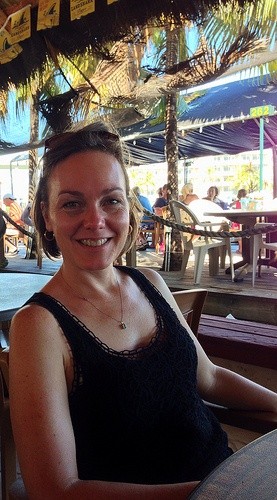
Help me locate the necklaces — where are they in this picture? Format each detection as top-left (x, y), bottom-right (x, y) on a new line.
top-left (60, 266), bottom-right (127, 329)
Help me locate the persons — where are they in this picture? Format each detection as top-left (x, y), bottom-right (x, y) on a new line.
top-left (3, 193), bottom-right (26, 256)
top-left (9, 126), bottom-right (276, 500)
top-left (0, 214), bottom-right (9, 269)
top-left (151, 184), bottom-right (249, 254)
top-left (131, 187), bottom-right (153, 252)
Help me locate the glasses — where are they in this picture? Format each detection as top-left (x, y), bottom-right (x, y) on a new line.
top-left (44, 131), bottom-right (119, 155)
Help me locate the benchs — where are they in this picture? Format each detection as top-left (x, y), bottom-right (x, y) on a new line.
top-left (197, 314), bottom-right (277, 394)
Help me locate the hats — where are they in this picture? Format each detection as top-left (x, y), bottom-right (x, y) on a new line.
top-left (3, 193), bottom-right (16, 200)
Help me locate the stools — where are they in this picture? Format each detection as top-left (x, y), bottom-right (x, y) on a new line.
top-left (3, 236), bottom-right (19, 253)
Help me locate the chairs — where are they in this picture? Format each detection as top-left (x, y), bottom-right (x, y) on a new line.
top-left (169, 200), bottom-right (277, 285)
top-left (140, 208), bottom-right (163, 248)
top-left (0, 288), bottom-right (208, 500)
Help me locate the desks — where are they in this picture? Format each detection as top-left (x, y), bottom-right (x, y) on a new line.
top-left (188, 427), bottom-right (276, 500)
top-left (203, 211), bottom-right (277, 282)
